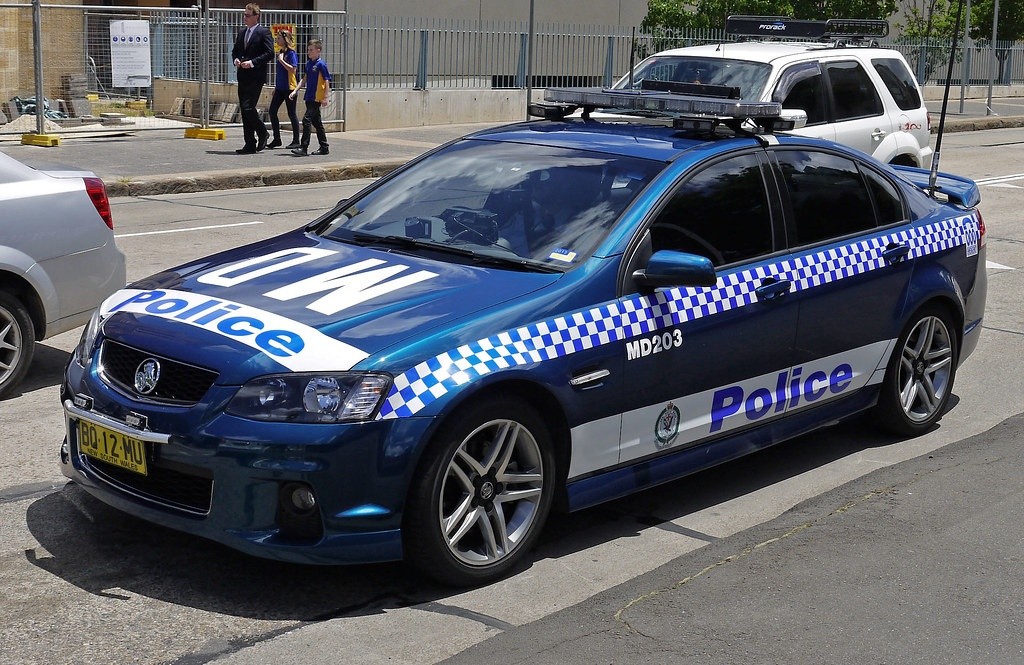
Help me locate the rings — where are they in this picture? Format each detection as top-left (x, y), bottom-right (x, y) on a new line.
top-left (245, 64), bottom-right (247, 65)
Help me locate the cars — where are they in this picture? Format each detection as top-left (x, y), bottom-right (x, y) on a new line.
top-left (59, 83), bottom-right (988, 588)
top-left (0, 151), bottom-right (127, 399)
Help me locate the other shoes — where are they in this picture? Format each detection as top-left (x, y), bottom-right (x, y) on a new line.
top-left (311, 147), bottom-right (329, 155)
top-left (286, 142), bottom-right (300, 149)
top-left (266, 139), bottom-right (282, 148)
top-left (236, 141), bottom-right (256, 154)
top-left (291, 148), bottom-right (307, 156)
top-left (256, 132), bottom-right (270, 151)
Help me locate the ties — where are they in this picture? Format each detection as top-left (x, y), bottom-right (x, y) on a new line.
top-left (244, 29), bottom-right (251, 49)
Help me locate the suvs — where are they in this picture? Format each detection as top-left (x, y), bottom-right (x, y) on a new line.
top-left (565, 16), bottom-right (933, 190)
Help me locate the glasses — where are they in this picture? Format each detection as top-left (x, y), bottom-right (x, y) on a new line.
top-left (245, 13), bottom-right (254, 17)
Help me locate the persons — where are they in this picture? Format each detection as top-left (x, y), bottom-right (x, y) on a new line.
top-left (499, 185), bottom-right (573, 254)
top-left (232, 3), bottom-right (275, 154)
top-left (289, 39), bottom-right (331, 156)
top-left (266, 29), bottom-right (301, 149)
